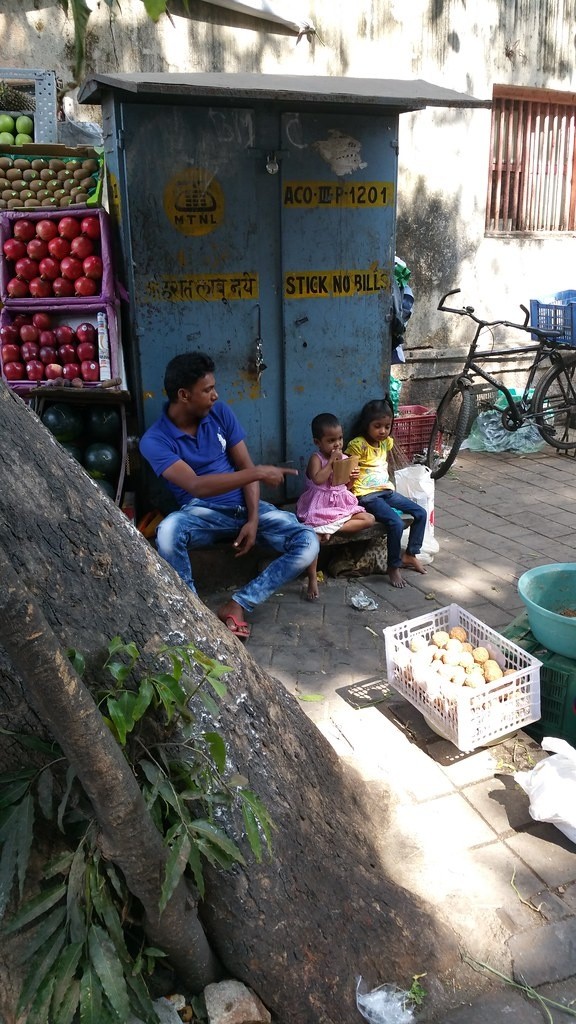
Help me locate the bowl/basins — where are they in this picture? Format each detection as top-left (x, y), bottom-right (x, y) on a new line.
top-left (517, 562), bottom-right (575, 659)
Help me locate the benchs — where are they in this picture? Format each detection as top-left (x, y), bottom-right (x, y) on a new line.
top-left (280, 504), bottom-right (415, 547)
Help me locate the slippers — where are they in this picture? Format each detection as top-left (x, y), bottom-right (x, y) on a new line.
top-left (222, 614), bottom-right (250, 638)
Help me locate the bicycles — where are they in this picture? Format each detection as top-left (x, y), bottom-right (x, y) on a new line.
top-left (426, 287), bottom-right (575, 481)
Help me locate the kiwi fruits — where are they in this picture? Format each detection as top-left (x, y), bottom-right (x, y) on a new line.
top-left (0, 154), bottom-right (101, 214)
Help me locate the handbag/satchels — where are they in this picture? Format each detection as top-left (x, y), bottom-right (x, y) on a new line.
top-left (393, 465), bottom-right (440, 566)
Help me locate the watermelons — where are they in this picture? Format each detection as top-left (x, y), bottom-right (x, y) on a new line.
top-left (29, 395), bottom-right (122, 504)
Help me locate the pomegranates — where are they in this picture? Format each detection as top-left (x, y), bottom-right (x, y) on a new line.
top-left (3, 216), bottom-right (104, 302)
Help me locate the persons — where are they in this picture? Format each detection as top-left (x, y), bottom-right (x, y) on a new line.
top-left (344, 391), bottom-right (428, 588)
top-left (295, 412), bottom-right (376, 599)
top-left (139, 350), bottom-right (321, 638)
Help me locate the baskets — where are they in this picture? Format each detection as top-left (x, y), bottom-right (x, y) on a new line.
top-left (390, 404), bottom-right (443, 462)
top-left (529, 289), bottom-right (576, 346)
top-left (381, 601), bottom-right (543, 752)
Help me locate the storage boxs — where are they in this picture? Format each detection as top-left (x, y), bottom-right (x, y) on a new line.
top-left (528, 289), bottom-right (576, 349)
top-left (498, 609), bottom-right (576, 749)
top-left (0, 141), bottom-right (106, 210)
top-left (0, 304), bottom-right (118, 388)
top-left (40, 393), bottom-right (128, 512)
top-left (391, 405), bottom-right (443, 463)
top-left (0, 209), bottom-right (117, 306)
top-left (383, 604), bottom-right (543, 753)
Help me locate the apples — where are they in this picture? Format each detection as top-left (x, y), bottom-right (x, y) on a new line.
top-left (1, 313), bottom-right (103, 383)
top-left (0, 110), bottom-right (32, 145)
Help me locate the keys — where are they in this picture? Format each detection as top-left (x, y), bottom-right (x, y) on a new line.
top-left (252, 338), bottom-right (267, 382)
